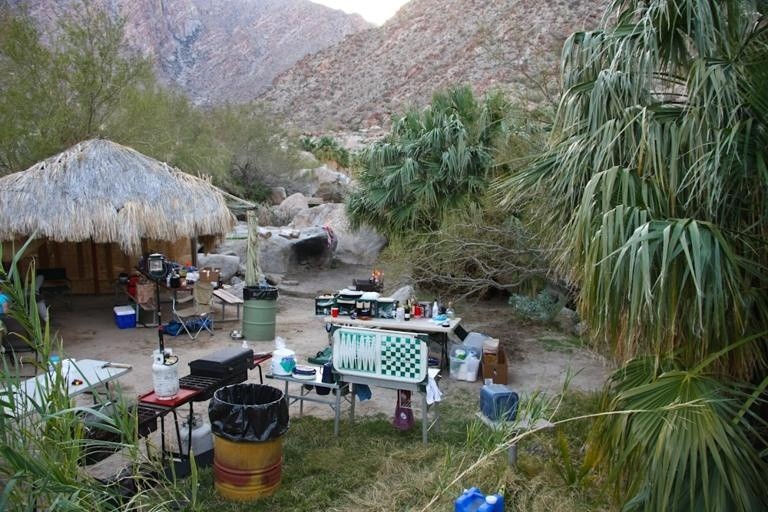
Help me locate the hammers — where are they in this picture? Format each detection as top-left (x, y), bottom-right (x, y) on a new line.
top-left (101, 361), bottom-right (132, 369)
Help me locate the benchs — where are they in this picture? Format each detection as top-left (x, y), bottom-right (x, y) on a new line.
top-left (116, 284), bottom-right (159, 327)
top-left (213, 288), bottom-right (243, 320)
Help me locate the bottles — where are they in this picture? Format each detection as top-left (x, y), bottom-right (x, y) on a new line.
top-left (405, 297), bottom-right (443, 322)
top-left (445, 301), bottom-right (455, 321)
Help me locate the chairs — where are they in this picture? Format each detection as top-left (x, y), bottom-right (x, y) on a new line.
top-left (169, 282), bottom-right (214, 340)
top-left (36, 266), bottom-right (72, 313)
top-left (0, 309), bottom-right (45, 378)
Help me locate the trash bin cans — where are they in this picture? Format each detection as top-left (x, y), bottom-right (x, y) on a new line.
top-left (242, 286), bottom-right (279, 341)
top-left (208, 384), bottom-right (289, 502)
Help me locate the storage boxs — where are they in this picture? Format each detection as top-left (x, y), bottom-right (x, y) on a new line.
top-left (113, 305), bottom-right (135, 328)
top-left (448, 332), bottom-right (511, 386)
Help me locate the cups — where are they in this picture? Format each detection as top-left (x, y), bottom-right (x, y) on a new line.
top-left (393, 308), bottom-right (404, 323)
top-left (49, 356), bottom-right (75, 388)
top-left (331, 308), bottom-right (339, 318)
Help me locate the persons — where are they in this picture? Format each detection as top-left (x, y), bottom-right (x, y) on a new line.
top-left (0, 285), bottom-right (12, 315)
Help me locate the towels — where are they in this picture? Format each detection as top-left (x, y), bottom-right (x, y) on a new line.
top-left (425, 373), bottom-right (441, 405)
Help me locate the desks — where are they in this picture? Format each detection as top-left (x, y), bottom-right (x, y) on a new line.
top-left (135, 263), bottom-right (192, 327)
top-left (0, 359), bottom-right (133, 424)
top-left (325, 312), bottom-right (463, 374)
top-left (324, 325), bottom-right (441, 442)
top-left (264, 365), bottom-right (357, 436)
